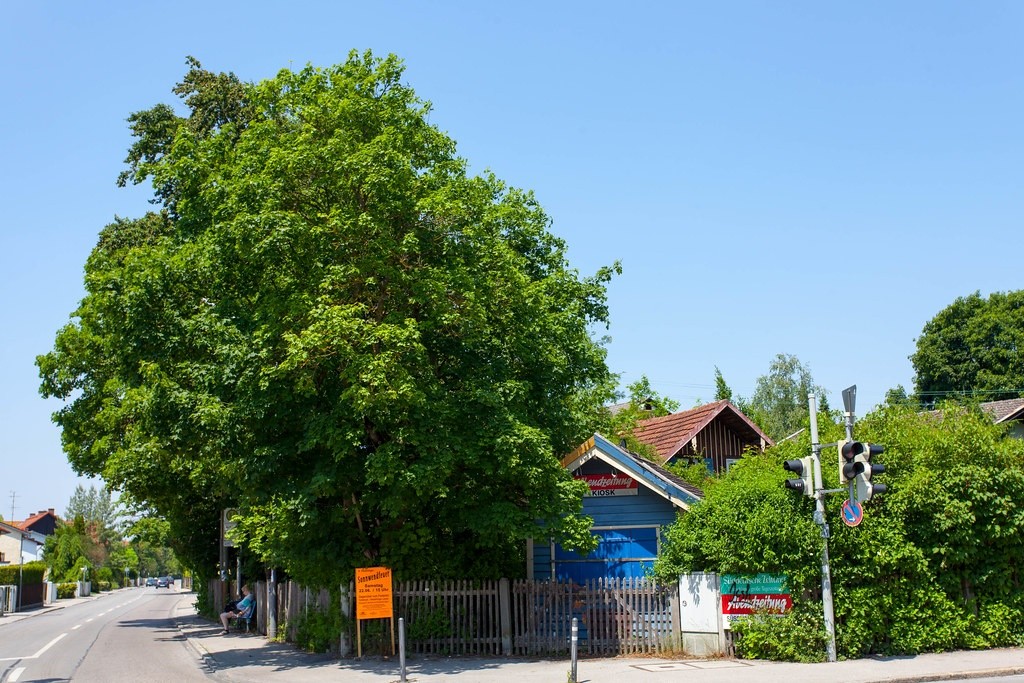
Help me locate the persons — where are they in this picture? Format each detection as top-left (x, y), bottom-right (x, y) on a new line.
top-left (220, 585), bottom-right (254, 636)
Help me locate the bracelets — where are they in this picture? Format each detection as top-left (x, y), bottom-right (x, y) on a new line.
top-left (240, 612), bottom-right (242, 614)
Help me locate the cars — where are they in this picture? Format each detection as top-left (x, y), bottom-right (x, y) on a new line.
top-left (146, 578), bottom-right (155, 587)
top-left (166, 575), bottom-right (175, 585)
top-left (156, 577), bottom-right (170, 589)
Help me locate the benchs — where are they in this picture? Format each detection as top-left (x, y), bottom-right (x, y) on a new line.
top-left (231, 601), bottom-right (257, 638)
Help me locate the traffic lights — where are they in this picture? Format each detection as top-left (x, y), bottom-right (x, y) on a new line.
top-left (783, 456), bottom-right (813, 496)
top-left (854, 442), bottom-right (888, 501)
top-left (837, 440), bottom-right (864, 484)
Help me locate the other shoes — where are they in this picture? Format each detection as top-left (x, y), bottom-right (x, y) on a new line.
top-left (219, 630), bottom-right (229, 635)
